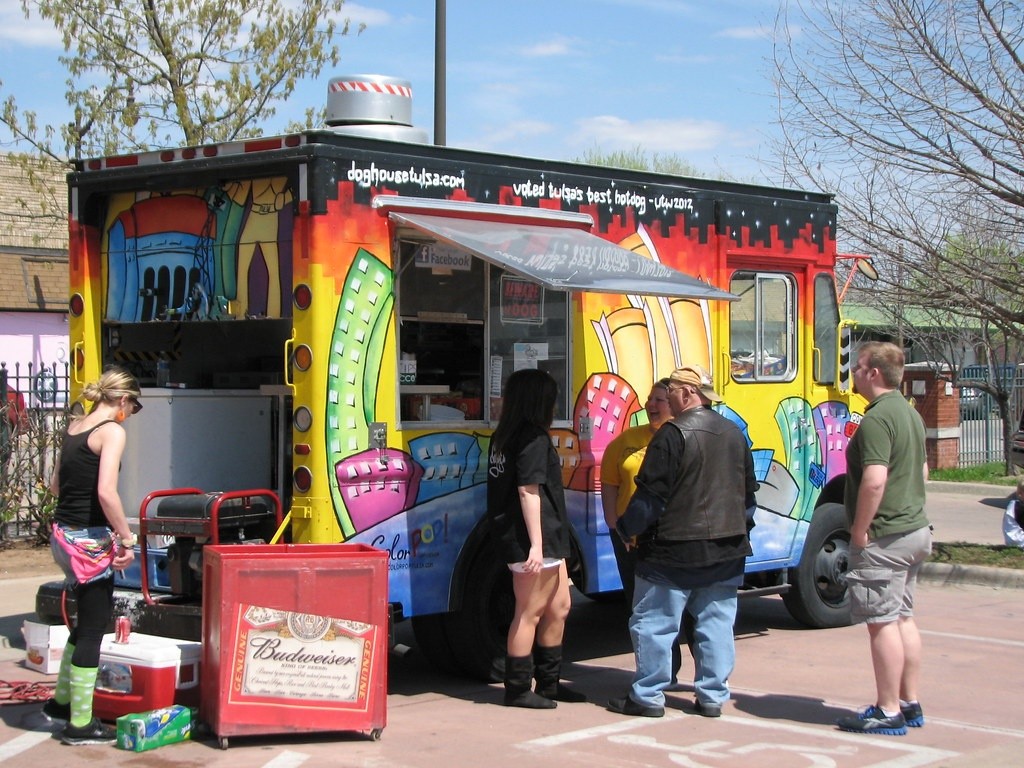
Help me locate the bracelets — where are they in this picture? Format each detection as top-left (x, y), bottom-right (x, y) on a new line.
top-left (121, 534), bottom-right (137, 548)
top-left (122, 545), bottom-right (135, 550)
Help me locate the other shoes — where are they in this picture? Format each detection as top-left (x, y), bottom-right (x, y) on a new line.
top-left (695, 695), bottom-right (721, 717)
top-left (607, 697), bottom-right (664, 716)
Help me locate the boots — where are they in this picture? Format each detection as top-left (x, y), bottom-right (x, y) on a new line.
top-left (534, 645), bottom-right (586, 703)
top-left (505, 654), bottom-right (557, 710)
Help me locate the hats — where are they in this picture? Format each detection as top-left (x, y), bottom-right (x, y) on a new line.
top-left (671, 364), bottom-right (723, 404)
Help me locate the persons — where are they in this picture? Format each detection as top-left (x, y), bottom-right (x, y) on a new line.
top-left (599, 379), bottom-right (694, 690)
top-left (487, 369), bottom-right (586, 708)
top-left (607, 364), bottom-right (757, 717)
top-left (835, 342), bottom-right (931, 736)
top-left (39, 368), bottom-right (139, 745)
top-left (1002, 480), bottom-right (1024, 548)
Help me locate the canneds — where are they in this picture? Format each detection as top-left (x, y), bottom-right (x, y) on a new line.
top-left (114, 616), bottom-right (131, 644)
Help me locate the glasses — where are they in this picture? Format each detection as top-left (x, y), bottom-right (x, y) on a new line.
top-left (666, 386), bottom-right (696, 396)
top-left (129, 395), bottom-right (144, 415)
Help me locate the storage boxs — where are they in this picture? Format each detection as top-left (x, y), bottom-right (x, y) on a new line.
top-left (20, 542), bottom-right (387, 751)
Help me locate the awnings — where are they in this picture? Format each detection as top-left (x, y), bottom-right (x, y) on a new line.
top-left (387, 212), bottom-right (742, 302)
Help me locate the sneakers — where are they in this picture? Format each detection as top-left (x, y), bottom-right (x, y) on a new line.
top-left (61, 719), bottom-right (119, 746)
top-left (837, 706), bottom-right (906, 735)
top-left (857, 702), bottom-right (924, 727)
top-left (40, 697), bottom-right (70, 724)
